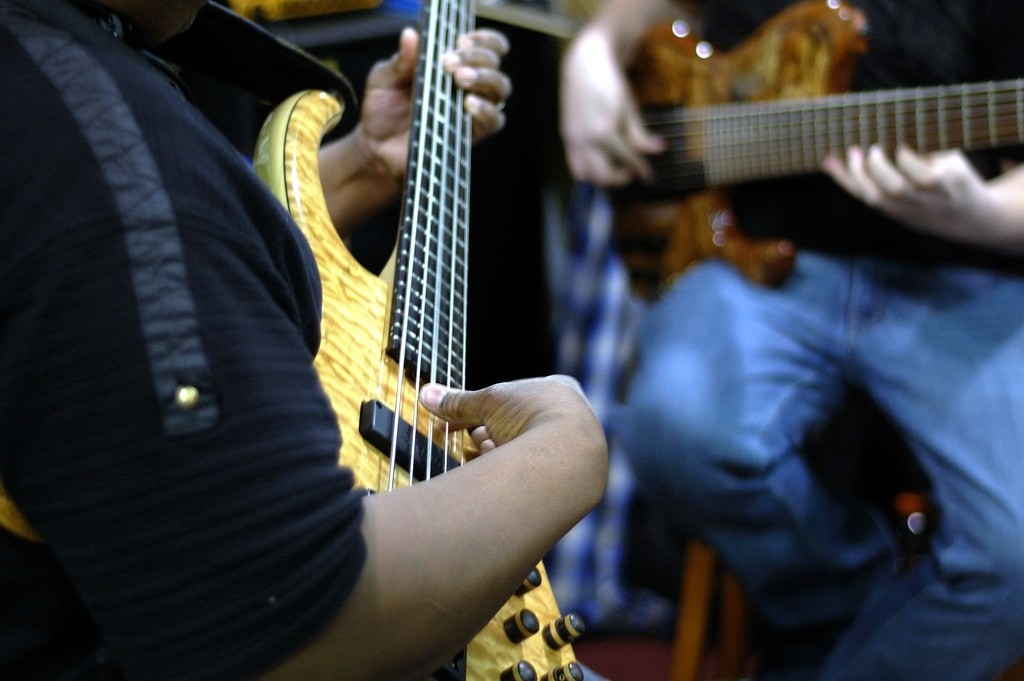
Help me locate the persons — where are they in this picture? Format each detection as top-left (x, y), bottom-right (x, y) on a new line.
top-left (560, 0), bottom-right (1024, 681)
top-left (0, 0), bottom-right (608, 681)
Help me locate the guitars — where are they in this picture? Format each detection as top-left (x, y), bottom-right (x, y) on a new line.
top-left (606, 0), bottom-right (1023, 304)
top-left (250, 0), bottom-right (589, 681)
